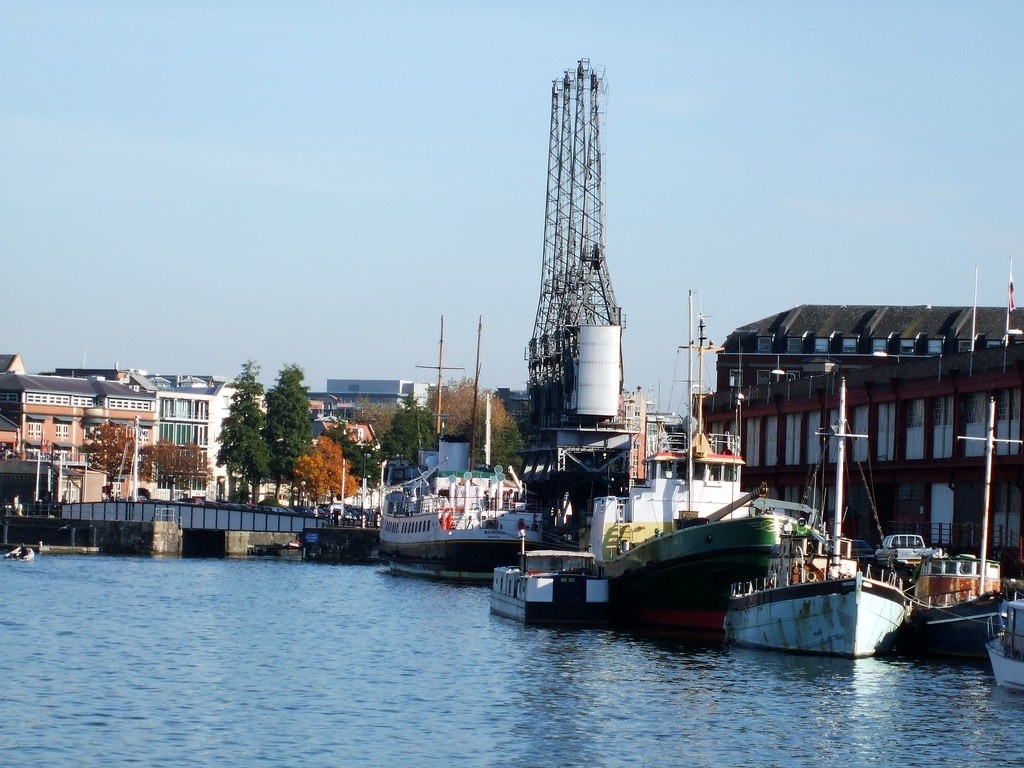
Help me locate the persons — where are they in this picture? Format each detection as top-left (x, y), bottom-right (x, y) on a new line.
top-left (328, 502), bottom-right (335, 523)
top-left (13, 494), bottom-right (19, 515)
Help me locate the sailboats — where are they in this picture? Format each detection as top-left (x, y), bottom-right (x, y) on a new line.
top-left (721, 374), bottom-right (908, 660)
top-left (895, 397), bottom-right (1024, 658)
top-left (587, 290), bottom-right (825, 636)
top-left (376, 313), bottom-right (555, 583)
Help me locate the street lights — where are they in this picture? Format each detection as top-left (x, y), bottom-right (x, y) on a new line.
top-left (361, 452), bottom-right (372, 528)
top-left (301, 480), bottom-right (306, 508)
top-left (219, 478), bottom-right (225, 501)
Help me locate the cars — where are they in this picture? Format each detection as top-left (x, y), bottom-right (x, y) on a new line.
top-left (850, 538), bottom-right (876, 570)
top-left (177, 496), bottom-right (381, 528)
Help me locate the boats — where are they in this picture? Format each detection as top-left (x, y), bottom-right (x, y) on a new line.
top-left (983, 588), bottom-right (1024, 691)
top-left (488, 527), bottom-right (612, 627)
top-left (3, 546), bottom-right (36, 563)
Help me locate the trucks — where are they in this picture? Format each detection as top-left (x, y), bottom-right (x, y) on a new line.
top-left (873, 534), bottom-right (938, 567)
